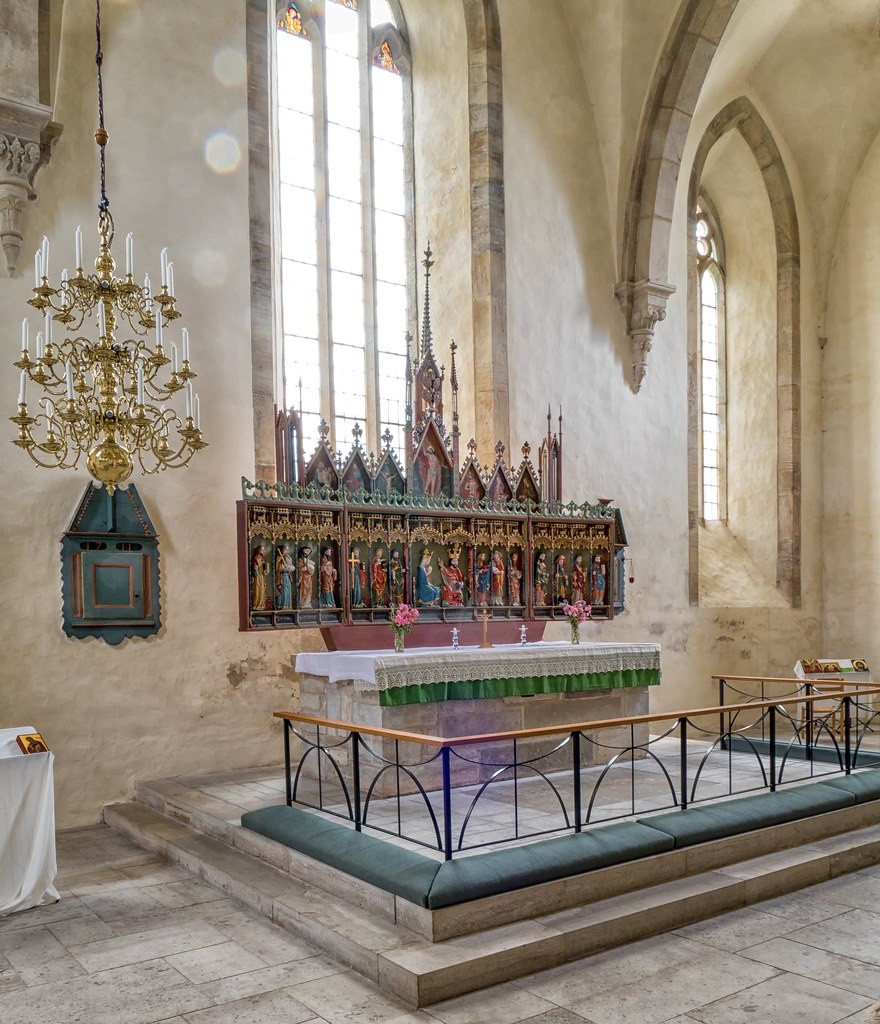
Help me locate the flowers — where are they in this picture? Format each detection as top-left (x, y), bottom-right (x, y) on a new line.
top-left (563, 599), bottom-right (593, 643)
top-left (388, 604), bottom-right (419, 649)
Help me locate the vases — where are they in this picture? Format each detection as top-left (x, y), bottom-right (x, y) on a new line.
top-left (394, 629), bottom-right (405, 653)
top-left (571, 624), bottom-right (579, 645)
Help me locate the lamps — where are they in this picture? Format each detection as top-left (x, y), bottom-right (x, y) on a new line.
top-left (8, 0), bottom-right (209, 497)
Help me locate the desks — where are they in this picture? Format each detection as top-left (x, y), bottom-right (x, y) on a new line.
top-left (0, 728), bottom-right (60, 912)
top-left (795, 658), bottom-right (873, 737)
top-left (295, 640), bottom-right (663, 797)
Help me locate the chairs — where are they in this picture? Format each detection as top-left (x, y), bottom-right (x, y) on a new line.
top-left (800, 677), bottom-right (845, 743)
